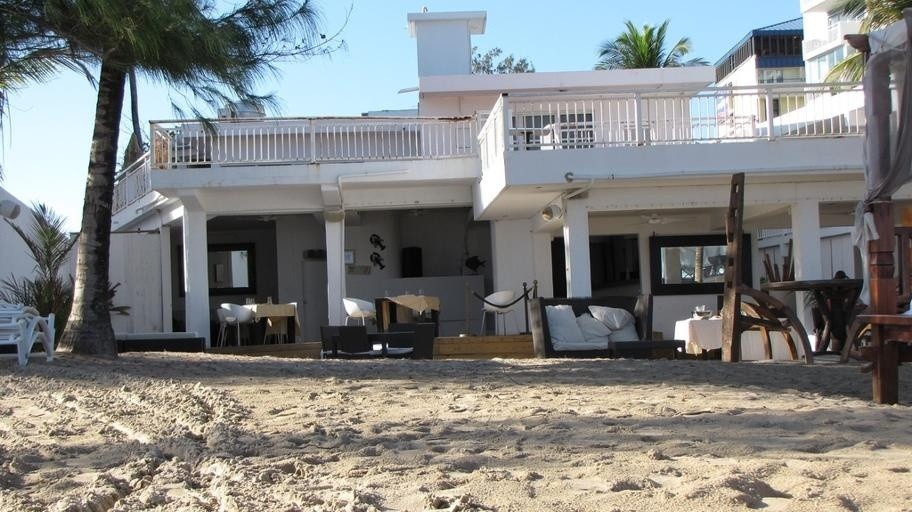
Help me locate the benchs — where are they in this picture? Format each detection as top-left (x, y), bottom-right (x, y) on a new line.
top-left (527, 293), bottom-right (653, 358)
top-left (609, 341), bottom-right (685, 359)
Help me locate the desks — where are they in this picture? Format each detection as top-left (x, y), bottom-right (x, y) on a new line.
top-left (375, 296), bottom-right (441, 336)
top-left (675, 317), bottom-right (796, 358)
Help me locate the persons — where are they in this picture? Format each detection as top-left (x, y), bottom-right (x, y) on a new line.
top-left (815, 270), bottom-right (846, 353)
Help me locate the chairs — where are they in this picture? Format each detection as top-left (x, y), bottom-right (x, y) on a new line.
top-left (321, 323), bottom-right (436, 360)
top-left (342, 297), bottom-right (377, 326)
top-left (0, 300), bottom-right (56, 366)
top-left (218, 302), bottom-right (298, 347)
top-left (481, 290), bottom-right (521, 336)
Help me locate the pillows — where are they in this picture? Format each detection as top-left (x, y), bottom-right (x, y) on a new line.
top-left (545, 304), bottom-right (639, 352)
